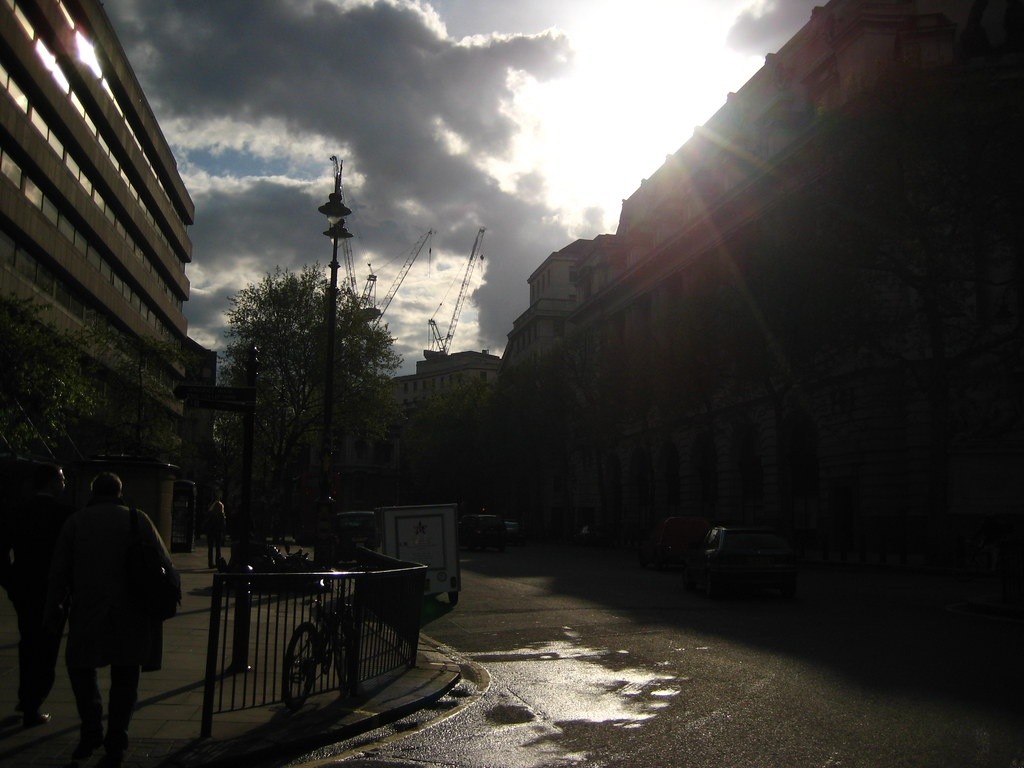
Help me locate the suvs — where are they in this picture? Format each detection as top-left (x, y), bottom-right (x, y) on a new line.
top-left (686, 522), bottom-right (800, 600)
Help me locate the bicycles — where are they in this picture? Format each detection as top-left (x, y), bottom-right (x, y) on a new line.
top-left (280, 579), bottom-right (358, 711)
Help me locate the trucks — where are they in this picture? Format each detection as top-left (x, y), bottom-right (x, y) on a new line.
top-left (373, 503), bottom-right (463, 608)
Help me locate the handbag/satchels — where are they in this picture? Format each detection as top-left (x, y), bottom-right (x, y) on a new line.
top-left (127, 540), bottom-right (180, 621)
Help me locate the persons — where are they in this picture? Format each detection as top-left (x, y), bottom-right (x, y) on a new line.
top-left (0, 458), bottom-right (81, 729)
top-left (46, 470), bottom-right (180, 762)
top-left (205, 500), bottom-right (226, 569)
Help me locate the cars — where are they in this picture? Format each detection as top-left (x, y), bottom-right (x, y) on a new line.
top-left (639, 515), bottom-right (713, 570)
top-left (336, 510), bottom-right (379, 551)
top-left (456, 511), bottom-right (525, 552)
top-left (570, 524), bottom-right (612, 548)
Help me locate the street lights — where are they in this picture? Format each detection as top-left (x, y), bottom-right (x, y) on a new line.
top-left (315, 172), bottom-right (355, 567)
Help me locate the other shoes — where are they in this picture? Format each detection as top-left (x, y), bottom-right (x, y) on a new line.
top-left (23, 716), bottom-right (47, 729)
top-left (98, 755), bottom-right (121, 768)
top-left (71, 731), bottom-right (102, 759)
top-left (14, 702), bottom-right (24, 713)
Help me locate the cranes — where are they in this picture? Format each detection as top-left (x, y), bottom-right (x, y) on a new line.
top-left (425, 320), bottom-right (450, 354)
top-left (444, 226), bottom-right (488, 356)
top-left (334, 228), bottom-right (437, 332)
